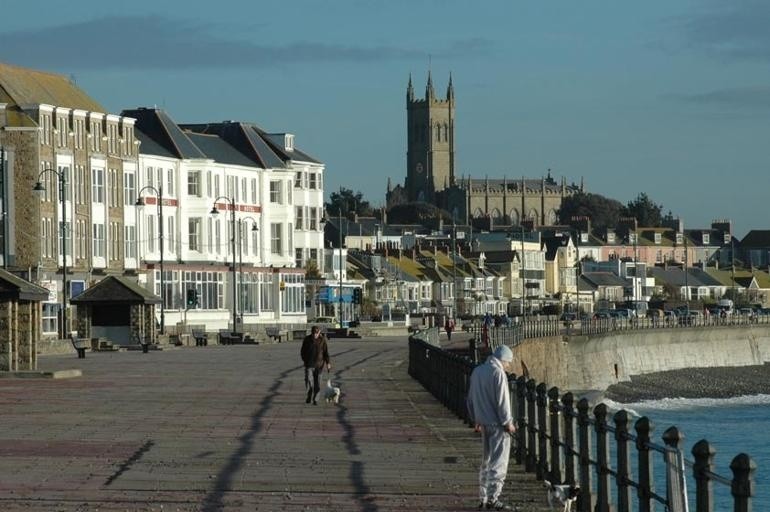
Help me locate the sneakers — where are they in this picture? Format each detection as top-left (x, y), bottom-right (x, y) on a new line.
top-left (477, 498), bottom-right (511, 509)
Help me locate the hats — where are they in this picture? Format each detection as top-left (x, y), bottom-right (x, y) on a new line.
top-left (311, 326), bottom-right (319, 334)
top-left (493, 345), bottom-right (513, 362)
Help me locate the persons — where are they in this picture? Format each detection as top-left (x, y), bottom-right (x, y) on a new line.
top-left (435, 316), bottom-right (441, 336)
top-left (702, 307), bottom-right (711, 326)
top-left (454, 308), bottom-right (519, 363)
top-left (721, 307), bottom-right (727, 325)
top-left (466, 345), bottom-right (516, 512)
top-left (444, 316), bottom-right (455, 340)
top-left (301, 326), bottom-right (331, 406)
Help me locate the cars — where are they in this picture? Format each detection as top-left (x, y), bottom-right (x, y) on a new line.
top-left (561, 299), bottom-right (766, 327)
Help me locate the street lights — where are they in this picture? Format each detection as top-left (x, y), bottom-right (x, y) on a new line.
top-left (320, 201), bottom-right (343, 328)
top-left (31, 169), bottom-right (68, 338)
top-left (210, 196), bottom-right (258, 334)
top-left (187, 288), bottom-right (198, 310)
top-left (136, 183), bottom-right (165, 334)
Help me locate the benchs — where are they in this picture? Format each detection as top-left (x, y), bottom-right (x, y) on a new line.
top-left (68, 335), bottom-right (91, 360)
top-left (190, 327), bottom-right (241, 346)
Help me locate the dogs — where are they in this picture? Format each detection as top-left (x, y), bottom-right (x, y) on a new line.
top-left (324, 379), bottom-right (340, 404)
top-left (544, 480), bottom-right (581, 512)
